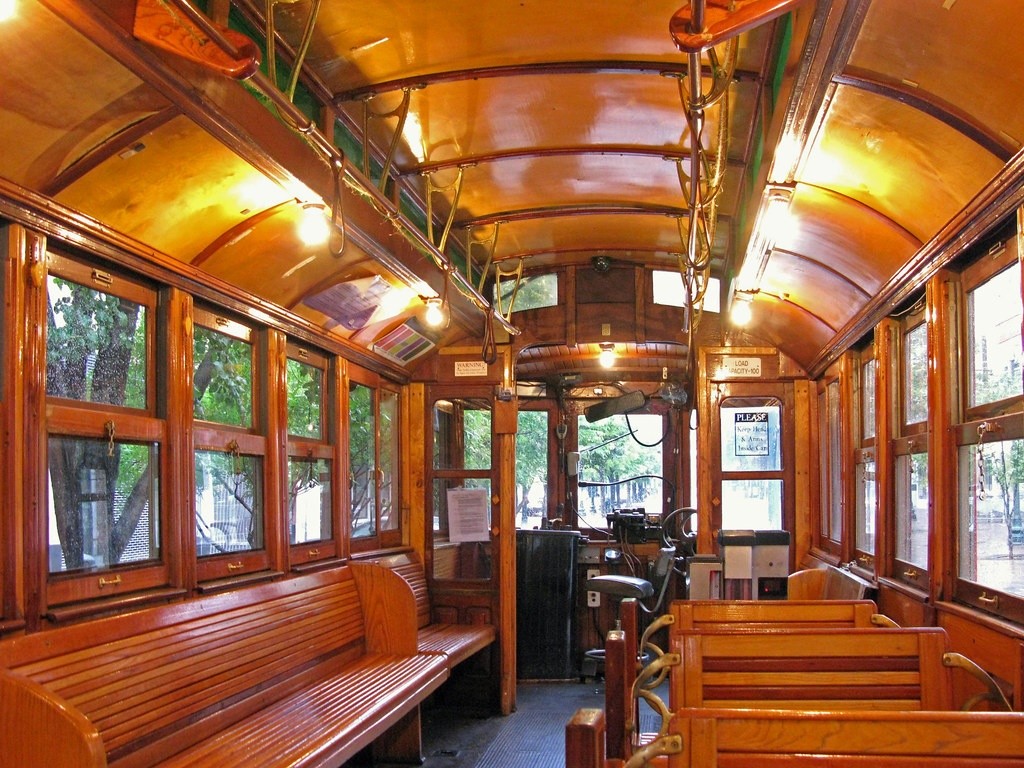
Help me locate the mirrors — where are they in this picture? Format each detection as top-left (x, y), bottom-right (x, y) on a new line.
top-left (584, 390), bottom-right (646, 423)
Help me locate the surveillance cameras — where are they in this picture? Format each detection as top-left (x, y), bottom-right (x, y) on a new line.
top-left (592, 257), bottom-right (610, 272)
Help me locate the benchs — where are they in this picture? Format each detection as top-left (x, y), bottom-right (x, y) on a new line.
top-left (0, 551), bottom-right (500, 768)
top-left (565, 567), bottom-right (1024, 768)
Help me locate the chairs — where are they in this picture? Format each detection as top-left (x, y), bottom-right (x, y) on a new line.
top-left (583, 547), bottom-right (678, 683)
